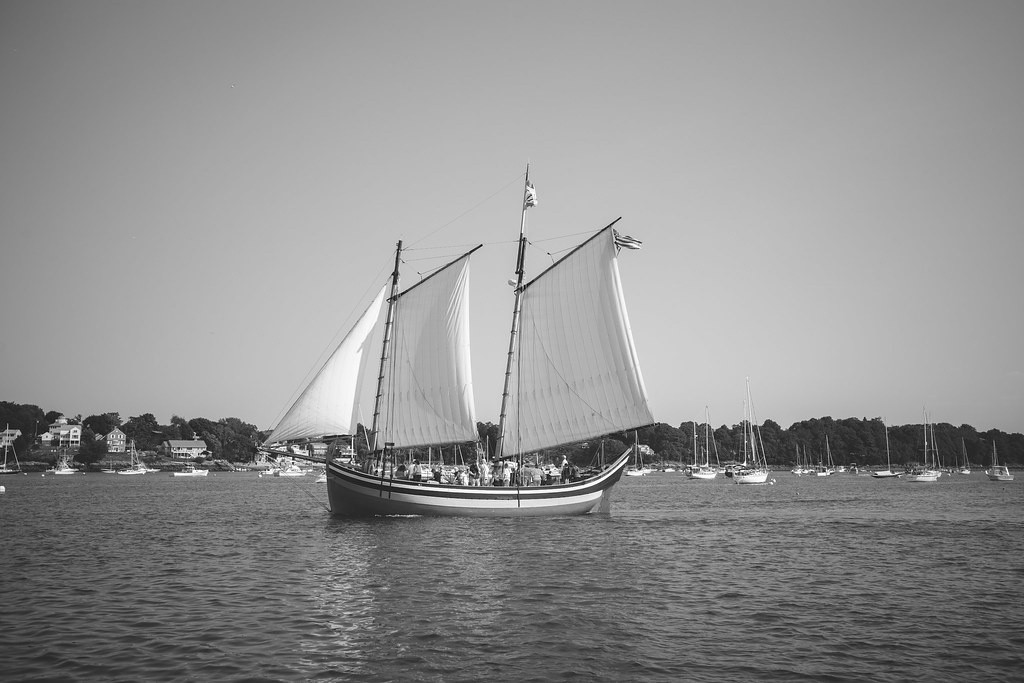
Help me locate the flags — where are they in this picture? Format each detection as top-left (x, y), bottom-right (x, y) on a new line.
top-left (613, 228), bottom-right (642, 250)
top-left (525, 184), bottom-right (537, 207)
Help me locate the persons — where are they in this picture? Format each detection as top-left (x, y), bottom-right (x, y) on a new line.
top-left (395, 455), bottom-right (580, 487)
top-left (737, 470), bottom-right (754, 475)
top-left (693, 468), bottom-right (701, 474)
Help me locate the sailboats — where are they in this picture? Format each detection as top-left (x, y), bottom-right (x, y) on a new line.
top-left (259, 159), bottom-right (654, 519)
top-left (0, 378), bottom-right (1015, 485)
